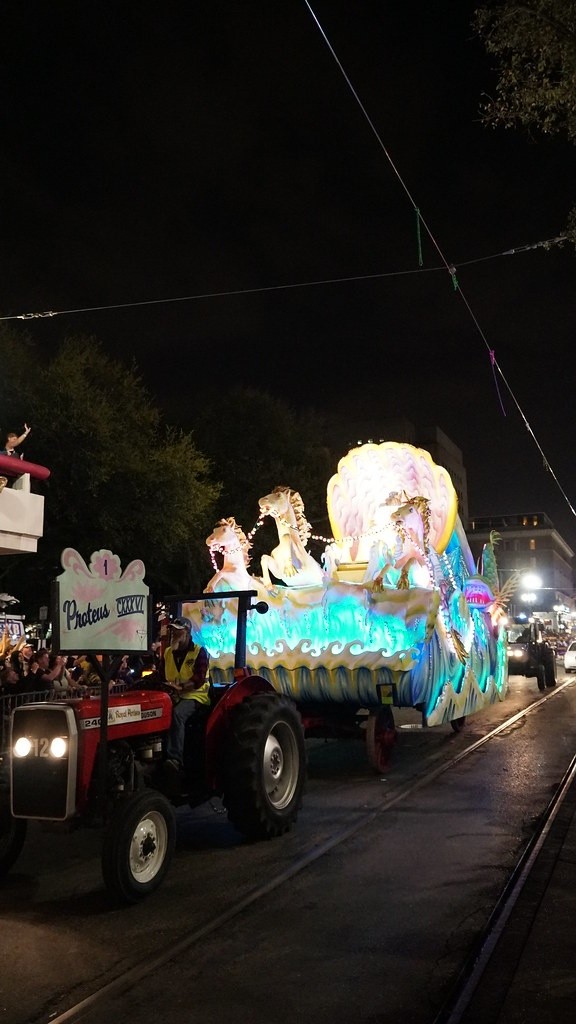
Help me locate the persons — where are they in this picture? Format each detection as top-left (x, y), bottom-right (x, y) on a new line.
top-left (0, 625), bottom-right (167, 722)
top-left (142, 616), bottom-right (208, 772)
top-left (539, 628), bottom-right (576, 655)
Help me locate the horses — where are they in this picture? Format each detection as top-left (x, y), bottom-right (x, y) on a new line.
top-left (376, 490), bottom-right (435, 589)
top-left (202, 516), bottom-right (265, 591)
top-left (258, 485), bottom-right (326, 587)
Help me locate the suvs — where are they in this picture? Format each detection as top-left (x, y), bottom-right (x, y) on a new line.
top-left (504, 615), bottom-right (558, 689)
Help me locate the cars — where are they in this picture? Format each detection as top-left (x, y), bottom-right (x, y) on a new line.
top-left (563, 641), bottom-right (576, 673)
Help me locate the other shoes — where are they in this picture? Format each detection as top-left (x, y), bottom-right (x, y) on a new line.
top-left (166, 759), bottom-right (179, 772)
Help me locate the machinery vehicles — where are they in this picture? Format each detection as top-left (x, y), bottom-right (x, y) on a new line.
top-left (1, 590), bottom-right (468, 906)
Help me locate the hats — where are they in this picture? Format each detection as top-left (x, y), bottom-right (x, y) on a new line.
top-left (166, 617), bottom-right (192, 633)
top-left (5, 644), bottom-right (17, 653)
